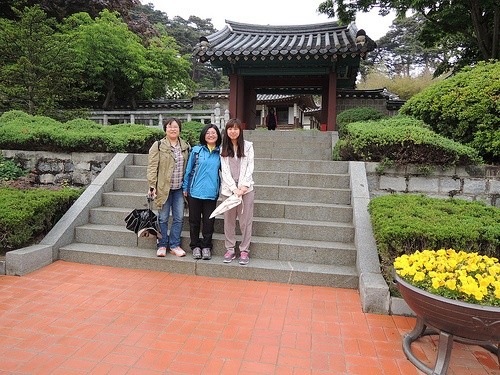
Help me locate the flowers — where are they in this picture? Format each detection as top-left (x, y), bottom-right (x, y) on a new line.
top-left (393, 248), bottom-right (500, 307)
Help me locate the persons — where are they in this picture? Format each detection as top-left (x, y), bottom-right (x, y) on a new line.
top-left (219, 118), bottom-right (254, 264)
top-left (265, 108), bottom-right (277, 130)
top-left (146, 118), bottom-right (191, 257)
top-left (183, 124), bottom-right (222, 259)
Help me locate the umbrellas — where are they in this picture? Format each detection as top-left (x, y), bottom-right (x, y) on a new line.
top-left (124, 187), bottom-right (162, 239)
top-left (209, 193), bottom-right (242, 218)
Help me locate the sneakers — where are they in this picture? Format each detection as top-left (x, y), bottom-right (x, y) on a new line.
top-left (202, 248), bottom-right (211, 260)
top-left (239, 252), bottom-right (249, 264)
top-left (168, 246), bottom-right (186, 256)
top-left (192, 247), bottom-right (201, 258)
top-left (223, 250), bottom-right (235, 263)
top-left (156, 247), bottom-right (166, 256)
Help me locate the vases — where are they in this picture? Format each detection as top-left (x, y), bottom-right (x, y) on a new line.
top-left (395, 274), bottom-right (500, 342)
